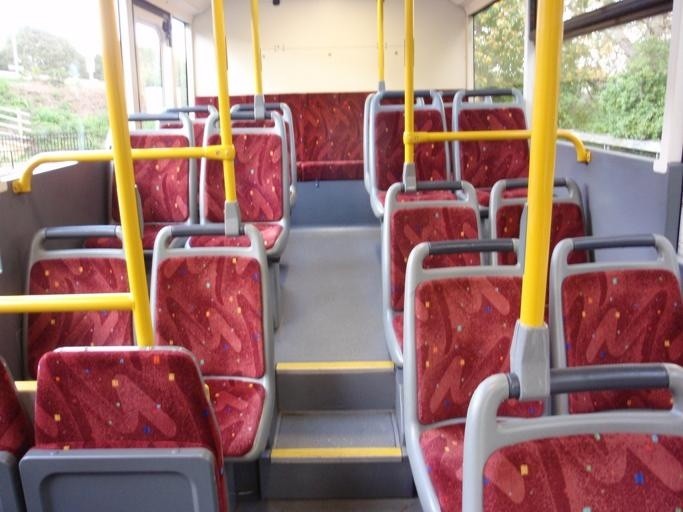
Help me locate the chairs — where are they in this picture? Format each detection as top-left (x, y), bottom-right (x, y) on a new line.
top-left (0, 91), bottom-right (683, 512)
